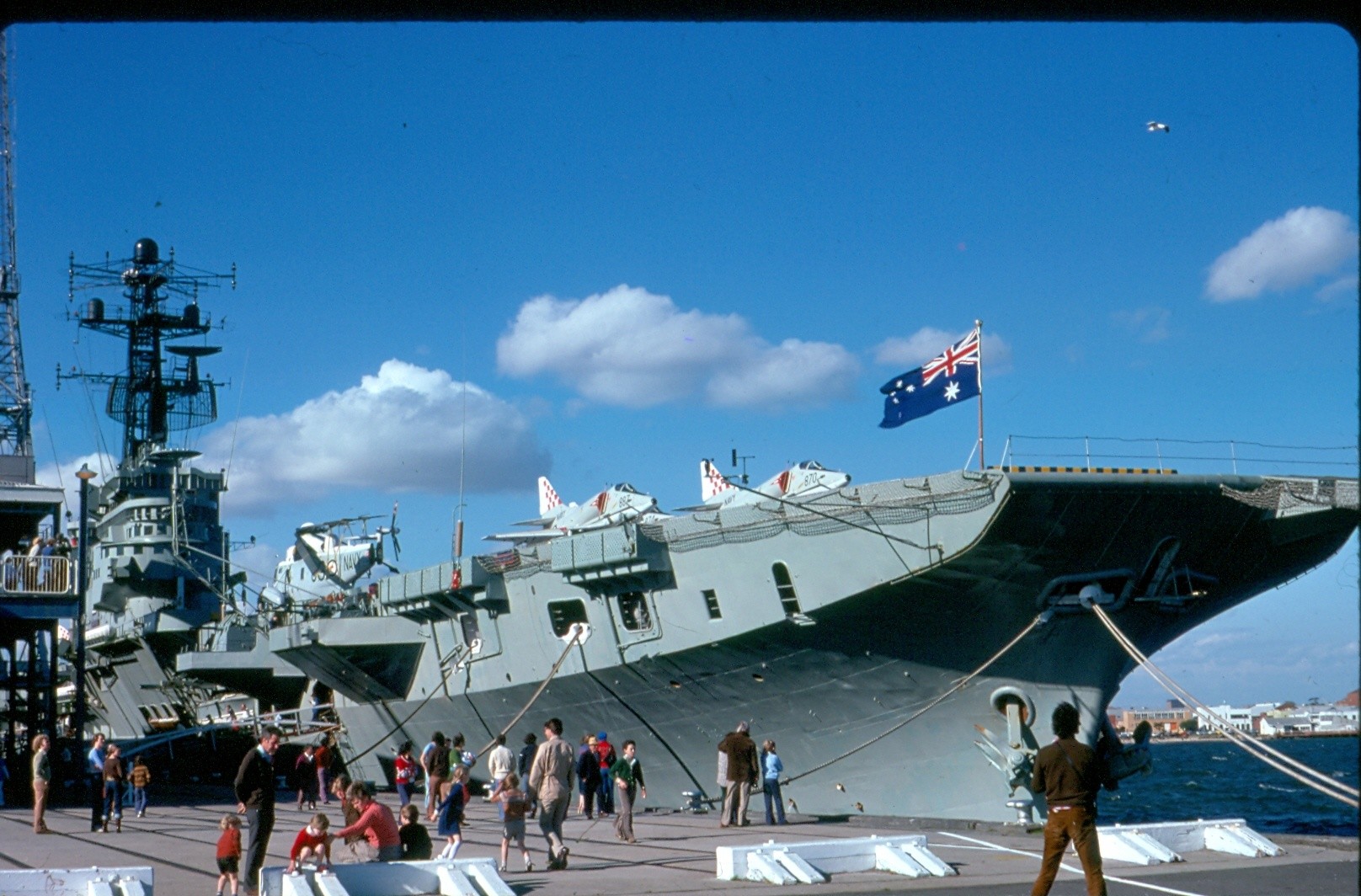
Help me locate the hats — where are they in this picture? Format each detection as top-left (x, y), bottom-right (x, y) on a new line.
top-left (599, 731), bottom-right (607, 740)
top-left (589, 736), bottom-right (600, 745)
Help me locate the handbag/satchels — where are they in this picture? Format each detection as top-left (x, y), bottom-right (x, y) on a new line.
top-left (1092, 794), bottom-right (1099, 818)
top-left (605, 752), bottom-right (617, 767)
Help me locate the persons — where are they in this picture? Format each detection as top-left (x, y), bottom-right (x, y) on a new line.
top-left (608, 740), bottom-right (646, 843)
top-left (285, 813), bottom-right (333, 876)
top-left (716, 732), bottom-right (740, 825)
top-left (527, 718), bottom-right (577, 872)
top-left (398, 805), bottom-right (432, 860)
top-left (101, 743), bottom-right (124, 832)
top-left (88, 733), bottom-right (104, 832)
top-left (1030, 702), bottom-right (1108, 896)
top-left (256, 595), bottom-right (332, 627)
top-left (717, 720), bottom-right (759, 826)
top-left (326, 781), bottom-right (402, 863)
top-left (32, 734), bottom-right (54, 833)
top-left (429, 764), bottom-right (469, 859)
top-left (489, 773), bottom-right (534, 872)
top-left (216, 815), bottom-right (241, 896)
top-left (331, 774), bottom-right (375, 864)
top-left (0, 533), bottom-right (71, 594)
top-left (0, 694), bottom-right (617, 822)
top-left (234, 726), bottom-right (283, 896)
top-left (760, 740), bottom-right (788, 825)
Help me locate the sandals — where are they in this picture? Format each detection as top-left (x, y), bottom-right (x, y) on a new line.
top-left (525, 860), bottom-right (532, 872)
top-left (497, 865), bottom-right (507, 871)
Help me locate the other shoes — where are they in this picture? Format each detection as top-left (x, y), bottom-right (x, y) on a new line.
top-left (92, 826), bottom-right (104, 832)
top-left (137, 814), bottom-right (143, 818)
top-left (617, 833), bottom-right (627, 841)
top-left (628, 836), bottom-right (636, 843)
top-left (138, 811), bottom-right (145, 816)
top-left (247, 889), bottom-right (258, 896)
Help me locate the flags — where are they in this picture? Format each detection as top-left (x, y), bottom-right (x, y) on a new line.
top-left (879, 328), bottom-right (980, 429)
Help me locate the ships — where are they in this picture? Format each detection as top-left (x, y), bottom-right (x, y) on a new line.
top-left (3, 237), bottom-right (1360, 826)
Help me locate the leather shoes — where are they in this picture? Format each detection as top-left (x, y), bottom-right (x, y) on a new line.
top-left (559, 848), bottom-right (570, 869)
top-left (547, 861), bottom-right (559, 869)
top-left (36, 829), bottom-right (54, 834)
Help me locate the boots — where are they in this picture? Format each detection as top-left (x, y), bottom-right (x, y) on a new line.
top-left (116, 818), bottom-right (121, 832)
top-left (103, 820), bottom-right (108, 833)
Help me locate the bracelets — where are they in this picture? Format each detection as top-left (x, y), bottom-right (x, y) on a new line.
top-left (642, 787), bottom-right (645, 789)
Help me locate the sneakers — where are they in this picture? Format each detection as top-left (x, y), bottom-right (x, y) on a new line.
top-left (316, 865), bottom-right (330, 874)
top-left (293, 866), bottom-right (303, 877)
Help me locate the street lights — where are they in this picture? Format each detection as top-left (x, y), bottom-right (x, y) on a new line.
top-left (74, 462), bottom-right (97, 805)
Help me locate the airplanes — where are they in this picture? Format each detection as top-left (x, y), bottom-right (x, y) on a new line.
top-left (672, 457), bottom-right (850, 512)
top-left (481, 475), bottom-right (658, 546)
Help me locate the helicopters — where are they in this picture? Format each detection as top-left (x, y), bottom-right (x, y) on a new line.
top-left (256, 501), bottom-right (403, 624)
